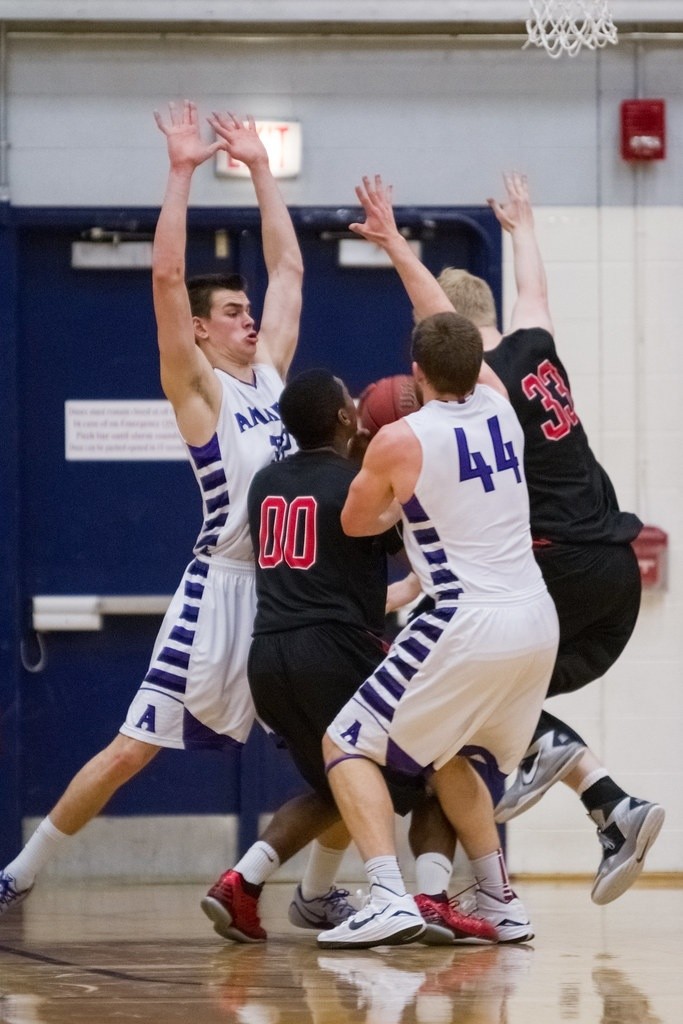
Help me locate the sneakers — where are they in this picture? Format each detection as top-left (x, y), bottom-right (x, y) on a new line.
top-left (493, 729), bottom-right (584, 823)
top-left (287, 885), bottom-right (357, 929)
top-left (316, 956), bottom-right (426, 1006)
top-left (316, 885), bottom-right (426, 949)
top-left (458, 890), bottom-right (535, 942)
top-left (414, 892), bottom-right (498, 945)
top-left (201, 868), bottom-right (268, 943)
top-left (0, 869), bottom-right (36, 917)
top-left (586, 796), bottom-right (665, 903)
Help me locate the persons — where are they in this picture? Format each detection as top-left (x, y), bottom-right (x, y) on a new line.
top-left (0, 950), bottom-right (650, 1024)
top-left (205, 171), bottom-right (664, 944)
top-left (0, 99), bottom-right (356, 931)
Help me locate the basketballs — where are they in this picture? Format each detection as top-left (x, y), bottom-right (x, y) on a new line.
top-left (356, 372), bottom-right (424, 436)
